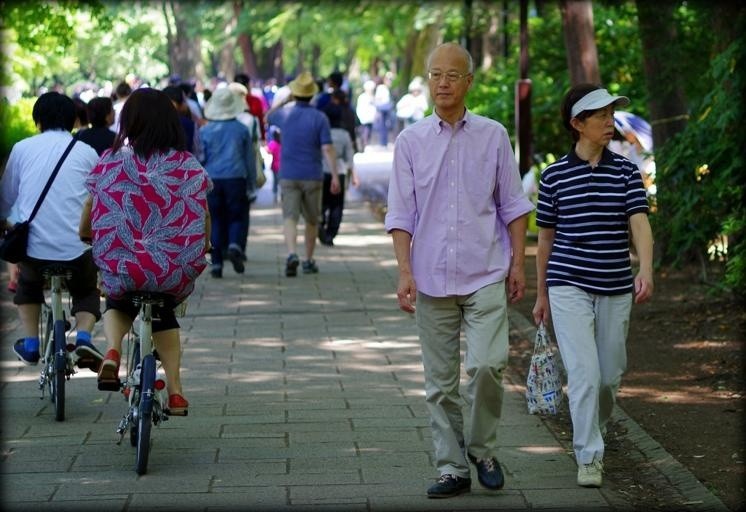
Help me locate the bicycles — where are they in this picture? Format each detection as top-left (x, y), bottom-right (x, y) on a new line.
top-left (97, 289), bottom-right (187, 476)
top-left (17, 267), bottom-right (98, 421)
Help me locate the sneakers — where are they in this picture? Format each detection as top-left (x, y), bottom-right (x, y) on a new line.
top-left (427, 474), bottom-right (471, 498)
top-left (226, 248), bottom-right (245, 273)
top-left (97, 349), bottom-right (120, 391)
top-left (467, 452), bottom-right (504, 490)
top-left (75, 339), bottom-right (104, 373)
top-left (303, 258), bottom-right (319, 273)
top-left (12, 338), bottom-right (39, 366)
top-left (166, 394), bottom-right (189, 415)
top-left (577, 459), bottom-right (606, 488)
top-left (286, 253), bottom-right (299, 276)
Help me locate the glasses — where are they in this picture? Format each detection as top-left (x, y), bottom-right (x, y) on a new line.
top-left (427, 70), bottom-right (471, 82)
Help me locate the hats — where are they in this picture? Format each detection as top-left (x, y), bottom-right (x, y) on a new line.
top-left (571, 89), bottom-right (630, 119)
top-left (288, 72), bottom-right (320, 97)
top-left (204, 87), bottom-right (246, 121)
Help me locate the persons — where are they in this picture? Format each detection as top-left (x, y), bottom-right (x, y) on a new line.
top-left (532, 82), bottom-right (654, 488)
top-left (79, 89), bottom-right (215, 417)
top-left (167, 70), bottom-right (367, 278)
top-left (1, 91), bottom-right (104, 372)
top-left (72, 81), bottom-right (135, 154)
top-left (354, 76), bottom-right (430, 152)
top-left (384, 43), bottom-right (537, 497)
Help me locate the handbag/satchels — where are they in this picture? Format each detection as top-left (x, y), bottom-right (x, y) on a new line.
top-left (252, 141), bottom-right (266, 188)
top-left (0, 221), bottom-right (28, 264)
top-left (526, 347), bottom-right (566, 416)
